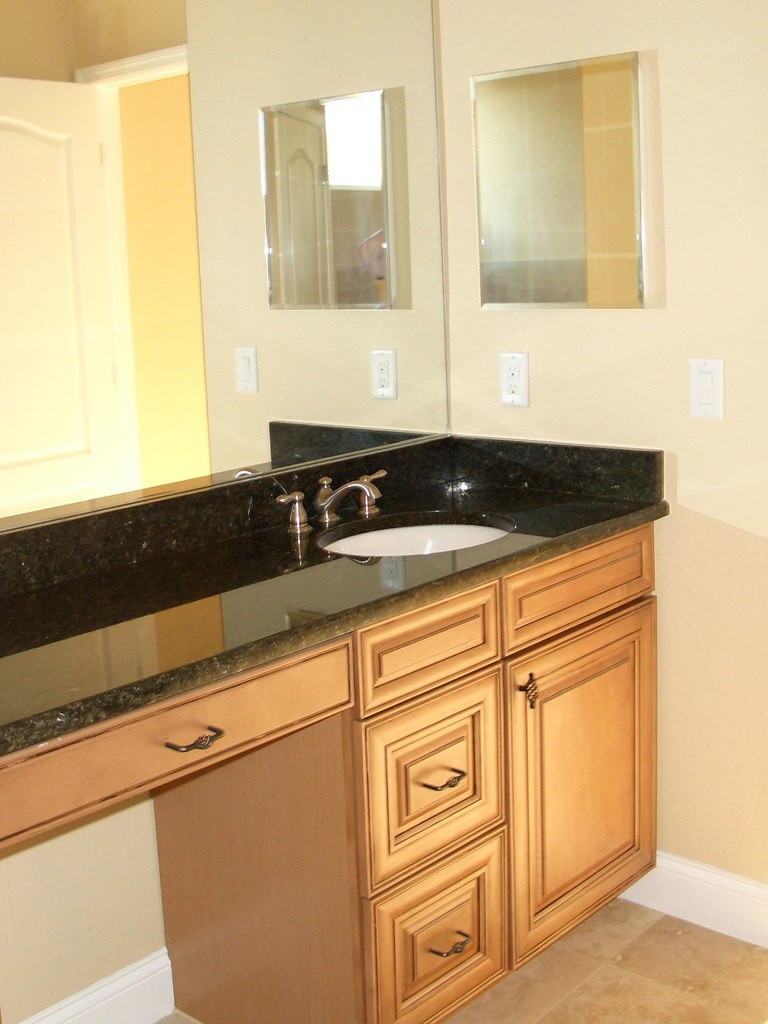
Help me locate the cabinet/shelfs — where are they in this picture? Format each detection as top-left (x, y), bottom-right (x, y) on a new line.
top-left (0, 521), bottom-right (656, 1024)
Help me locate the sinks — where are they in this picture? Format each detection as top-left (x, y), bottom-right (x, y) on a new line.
top-left (312, 506), bottom-right (519, 560)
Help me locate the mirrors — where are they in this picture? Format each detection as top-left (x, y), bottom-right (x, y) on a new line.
top-left (0, 0), bottom-right (451, 534)
top-left (469, 52), bottom-right (645, 310)
top-left (258, 86), bottom-right (411, 310)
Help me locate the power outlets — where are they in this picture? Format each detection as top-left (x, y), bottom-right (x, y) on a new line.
top-left (371, 349), bottom-right (398, 398)
top-left (500, 352), bottom-right (532, 408)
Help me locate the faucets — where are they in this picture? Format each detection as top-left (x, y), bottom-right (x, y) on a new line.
top-left (346, 555), bottom-right (382, 566)
top-left (314, 476), bottom-right (382, 522)
top-left (234, 466), bottom-right (258, 478)
